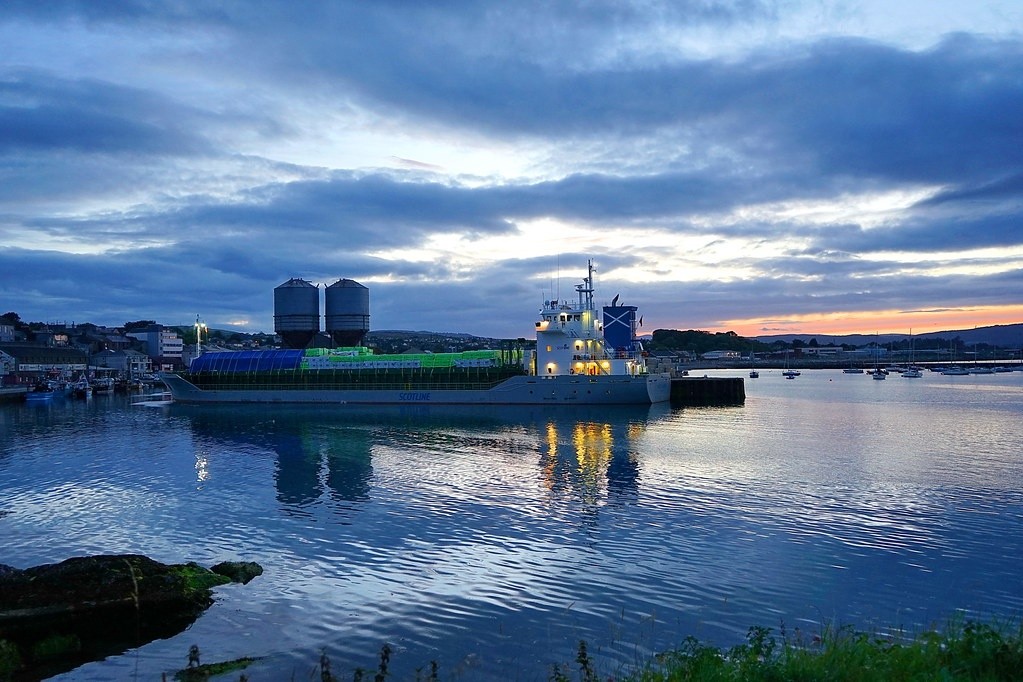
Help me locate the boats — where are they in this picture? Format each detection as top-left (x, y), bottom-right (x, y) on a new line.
top-left (0, 360), bottom-right (165, 401)
top-left (843, 369), bottom-right (865, 374)
top-left (749, 370), bottom-right (759, 378)
top-left (783, 370), bottom-right (801, 376)
top-left (786, 376), bottom-right (795, 380)
top-left (682, 370), bottom-right (689, 376)
top-left (866, 328), bottom-right (1023, 381)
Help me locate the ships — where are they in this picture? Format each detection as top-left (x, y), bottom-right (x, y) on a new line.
top-left (160, 256), bottom-right (672, 406)
